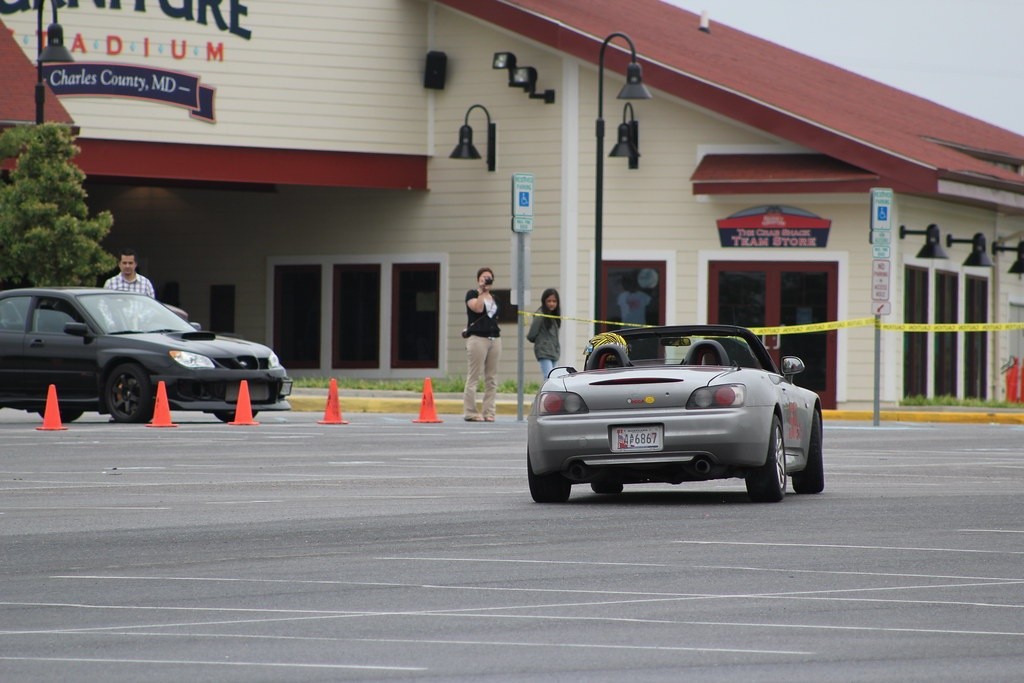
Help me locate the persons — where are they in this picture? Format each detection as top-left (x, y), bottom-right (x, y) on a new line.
top-left (462, 267), bottom-right (502, 423)
top-left (527, 288), bottom-right (561, 378)
top-left (104, 248), bottom-right (156, 301)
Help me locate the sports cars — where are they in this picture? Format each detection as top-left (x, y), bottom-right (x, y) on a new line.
top-left (525, 323), bottom-right (825, 504)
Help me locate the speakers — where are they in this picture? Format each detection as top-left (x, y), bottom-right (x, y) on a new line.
top-left (425, 51), bottom-right (447, 90)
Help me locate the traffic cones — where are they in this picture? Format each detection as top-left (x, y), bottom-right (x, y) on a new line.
top-left (315, 379), bottom-right (351, 425)
top-left (144, 380), bottom-right (180, 428)
top-left (227, 380), bottom-right (260, 425)
top-left (412, 377), bottom-right (445, 424)
top-left (34, 384), bottom-right (70, 430)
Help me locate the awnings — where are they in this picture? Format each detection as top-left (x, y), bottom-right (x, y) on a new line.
top-left (690, 153), bottom-right (881, 194)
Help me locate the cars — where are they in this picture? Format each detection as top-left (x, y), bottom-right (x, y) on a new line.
top-left (0, 285), bottom-right (295, 424)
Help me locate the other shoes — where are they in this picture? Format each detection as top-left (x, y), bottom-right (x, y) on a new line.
top-left (465, 416), bottom-right (486, 422)
top-left (484, 416), bottom-right (494, 422)
top-left (109, 415), bottom-right (115, 422)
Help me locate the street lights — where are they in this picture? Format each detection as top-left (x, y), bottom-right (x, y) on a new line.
top-left (594, 30), bottom-right (654, 339)
top-left (34, 0), bottom-right (76, 128)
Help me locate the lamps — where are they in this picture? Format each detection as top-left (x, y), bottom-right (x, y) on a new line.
top-left (946, 232), bottom-right (995, 268)
top-left (448, 104), bottom-right (498, 173)
top-left (514, 67), bottom-right (555, 105)
top-left (899, 223), bottom-right (949, 260)
top-left (491, 50), bottom-right (516, 87)
top-left (607, 102), bottom-right (642, 171)
top-left (991, 240), bottom-right (1024, 275)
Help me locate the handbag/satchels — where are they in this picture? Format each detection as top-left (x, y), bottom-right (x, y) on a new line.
top-left (462, 327), bottom-right (469, 338)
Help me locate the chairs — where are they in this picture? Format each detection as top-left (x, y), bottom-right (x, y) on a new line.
top-left (584, 343), bottom-right (632, 371)
top-left (682, 339), bottom-right (730, 366)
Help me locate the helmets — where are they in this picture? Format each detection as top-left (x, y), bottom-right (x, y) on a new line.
top-left (583, 332), bottom-right (628, 368)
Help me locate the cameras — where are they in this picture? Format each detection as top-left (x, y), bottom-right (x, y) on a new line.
top-left (484, 278), bottom-right (493, 286)
